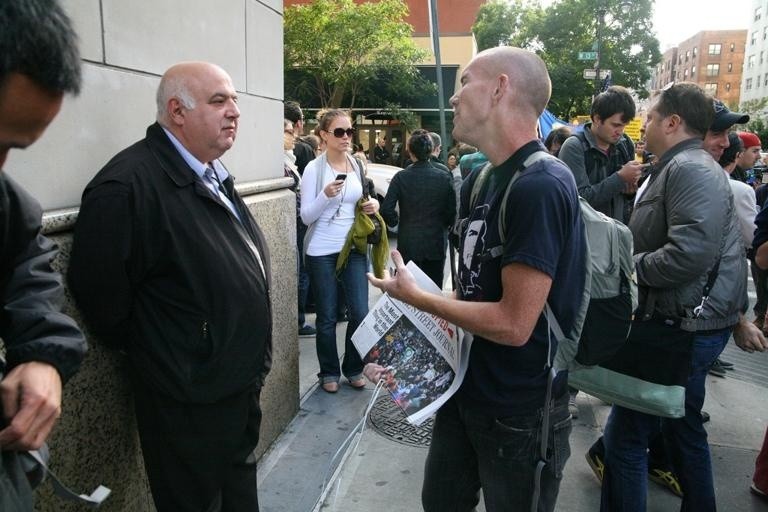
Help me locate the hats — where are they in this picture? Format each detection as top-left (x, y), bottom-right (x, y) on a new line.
top-left (707, 96), bottom-right (750, 131)
top-left (738, 133), bottom-right (760, 146)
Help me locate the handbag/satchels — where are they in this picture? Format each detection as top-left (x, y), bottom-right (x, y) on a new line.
top-left (621, 152), bottom-right (733, 387)
top-left (355, 157), bottom-right (383, 244)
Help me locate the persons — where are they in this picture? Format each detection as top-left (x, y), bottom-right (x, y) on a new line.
top-left (352, 143), bottom-right (368, 176)
top-left (452, 144), bottom-right (477, 178)
top-left (707, 134), bottom-right (761, 375)
top-left (381, 131), bottom-right (457, 291)
top-left (284, 102), bottom-right (315, 175)
top-left (406, 132), bottom-right (454, 255)
top-left (363, 45), bottom-right (587, 511)
top-left (635, 142), bottom-right (649, 160)
top-left (718, 130), bottom-right (756, 260)
top-left (374, 139), bottom-right (388, 164)
top-left (65, 60), bottom-right (274, 512)
top-left (545, 126), bottom-right (572, 154)
top-left (298, 107), bottom-right (388, 393)
top-left (557, 86), bottom-right (644, 421)
top-left (304, 135), bottom-right (319, 157)
top-left (447, 154), bottom-right (456, 170)
top-left (755, 241), bottom-right (767, 270)
top-left (584, 93), bottom-right (768, 500)
top-left (0, 0), bottom-right (89, 512)
top-left (314, 124), bottom-right (327, 152)
top-left (598, 81), bottom-right (745, 512)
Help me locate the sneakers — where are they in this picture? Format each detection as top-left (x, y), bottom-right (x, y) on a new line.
top-left (707, 357), bottom-right (735, 377)
top-left (648, 460), bottom-right (683, 498)
top-left (584, 438), bottom-right (605, 481)
top-left (297, 326), bottom-right (317, 338)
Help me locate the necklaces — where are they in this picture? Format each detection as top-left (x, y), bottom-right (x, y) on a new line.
top-left (326, 160), bottom-right (349, 227)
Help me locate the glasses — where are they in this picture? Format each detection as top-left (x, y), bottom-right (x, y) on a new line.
top-left (663, 81), bottom-right (680, 118)
top-left (326, 128), bottom-right (353, 138)
top-left (284, 129), bottom-right (294, 134)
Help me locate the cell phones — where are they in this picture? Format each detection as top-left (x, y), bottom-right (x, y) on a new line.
top-left (335, 173), bottom-right (348, 184)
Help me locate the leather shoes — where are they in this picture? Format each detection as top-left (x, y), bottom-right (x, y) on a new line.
top-left (319, 381), bottom-right (338, 392)
top-left (348, 378), bottom-right (366, 390)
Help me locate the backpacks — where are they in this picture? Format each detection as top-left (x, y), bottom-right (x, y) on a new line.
top-left (467, 151), bottom-right (638, 374)
